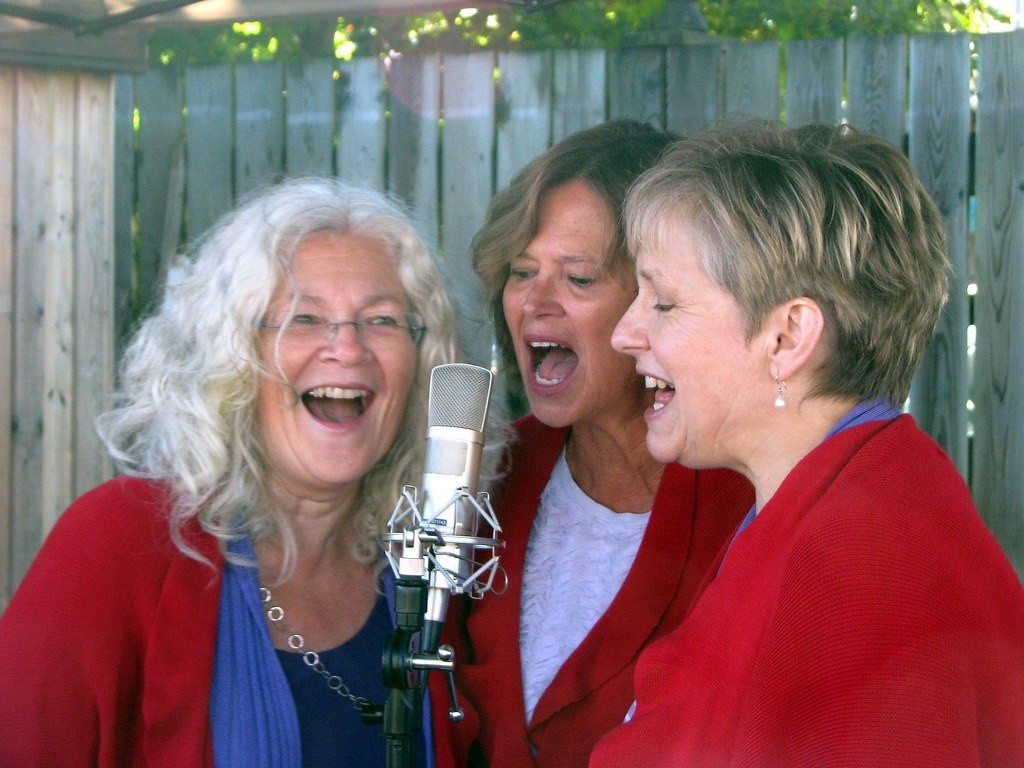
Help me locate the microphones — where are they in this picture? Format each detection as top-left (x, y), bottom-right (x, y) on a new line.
top-left (420, 363), bottom-right (493, 654)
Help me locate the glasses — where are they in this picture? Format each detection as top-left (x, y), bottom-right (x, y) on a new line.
top-left (260, 310), bottom-right (426, 347)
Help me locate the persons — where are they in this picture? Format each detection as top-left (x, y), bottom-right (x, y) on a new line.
top-left (456, 119), bottom-right (756, 768)
top-left (589, 111), bottom-right (1024, 768)
top-left (0, 176), bottom-right (465, 768)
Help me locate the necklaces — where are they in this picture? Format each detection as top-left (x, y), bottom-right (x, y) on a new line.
top-left (258, 586), bottom-right (374, 712)
